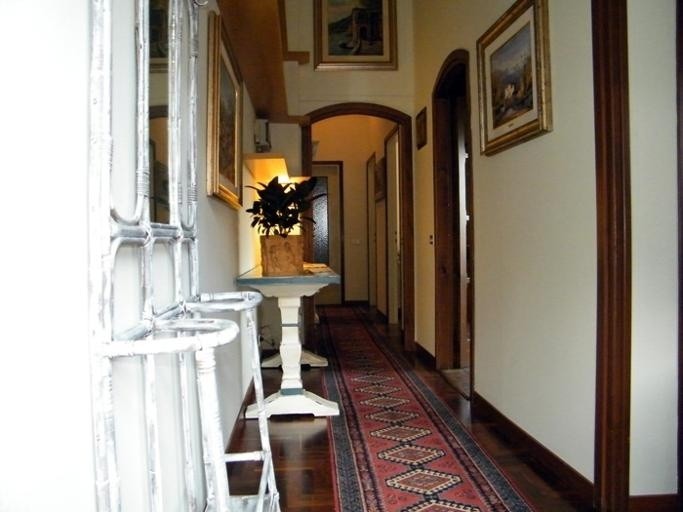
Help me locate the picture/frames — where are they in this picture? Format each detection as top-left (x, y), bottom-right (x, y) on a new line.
top-left (311, 0), bottom-right (400, 72)
top-left (475, 0), bottom-right (554, 156)
top-left (416, 106), bottom-right (427, 149)
top-left (374, 158), bottom-right (385, 202)
top-left (207, 9), bottom-right (244, 212)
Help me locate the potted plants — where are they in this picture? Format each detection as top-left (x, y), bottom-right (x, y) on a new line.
top-left (244, 174), bottom-right (328, 277)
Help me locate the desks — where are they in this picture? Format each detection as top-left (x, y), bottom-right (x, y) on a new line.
top-left (235, 263), bottom-right (343, 417)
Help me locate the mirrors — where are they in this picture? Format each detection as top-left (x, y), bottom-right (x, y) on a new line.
top-left (147, 1), bottom-right (174, 226)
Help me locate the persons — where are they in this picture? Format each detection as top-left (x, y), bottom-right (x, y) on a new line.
top-left (338, 1), bottom-right (382, 56)
